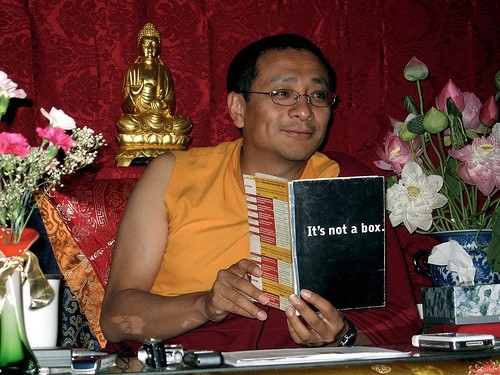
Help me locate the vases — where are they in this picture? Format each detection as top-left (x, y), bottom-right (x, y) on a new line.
top-left (425, 228), bottom-right (500, 287)
top-left (0, 227), bottom-right (40, 375)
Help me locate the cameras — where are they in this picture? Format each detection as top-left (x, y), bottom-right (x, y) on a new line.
top-left (140, 337), bottom-right (224, 370)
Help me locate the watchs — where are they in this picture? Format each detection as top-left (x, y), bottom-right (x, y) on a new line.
top-left (334, 316), bottom-right (358, 347)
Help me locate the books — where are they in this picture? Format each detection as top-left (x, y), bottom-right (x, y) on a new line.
top-left (242, 173), bottom-right (387, 317)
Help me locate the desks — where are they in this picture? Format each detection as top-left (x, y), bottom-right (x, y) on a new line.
top-left (92, 344), bottom-right (500, 375)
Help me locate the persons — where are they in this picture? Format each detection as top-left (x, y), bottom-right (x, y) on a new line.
top-left (98, 32), bottom-right (424, 357)
top-left (115, 24), bottom-right (193, 135)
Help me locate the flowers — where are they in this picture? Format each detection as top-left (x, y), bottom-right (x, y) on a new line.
top-left (0, 69), bottom-right (108, 244)
top-left (373, 56), bottom-right (500, 235)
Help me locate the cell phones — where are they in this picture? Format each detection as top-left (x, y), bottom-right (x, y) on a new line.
top-left (411, 333), bottom-right (495, 351)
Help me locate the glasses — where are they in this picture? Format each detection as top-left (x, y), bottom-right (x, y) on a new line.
top-left (235, 88), bottom-right (335, 108)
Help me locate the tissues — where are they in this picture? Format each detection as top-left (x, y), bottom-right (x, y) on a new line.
top-left (419, 239), bottom-right (500, 325)
